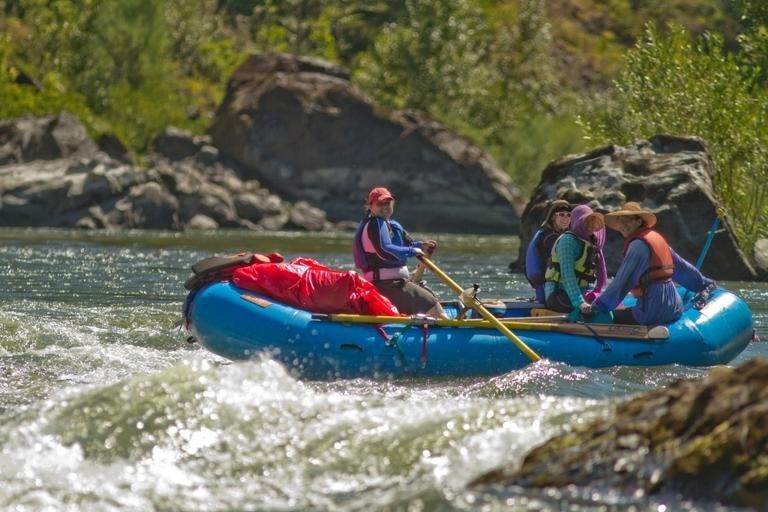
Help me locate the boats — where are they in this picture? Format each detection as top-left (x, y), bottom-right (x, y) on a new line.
top-left (183, 252), bottom-right (755, 381)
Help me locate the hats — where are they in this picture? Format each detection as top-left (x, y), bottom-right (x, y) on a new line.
top-left (552, 199), bottom-right (657, 231)
top-left (368, 188), bottom-right (394, 205)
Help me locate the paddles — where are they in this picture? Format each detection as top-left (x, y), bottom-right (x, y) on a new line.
top-left (310, 313), bottom-right (669, 339)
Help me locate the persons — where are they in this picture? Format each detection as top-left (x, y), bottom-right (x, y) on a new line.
top-left (590, 199), bottom-right (717, 327)
top-left (524, 199), bottom-right (572, 303)
top-left (350, 187), bottom-right (451, 319)
top-left (544, 204), bottom-right (624, 316)
